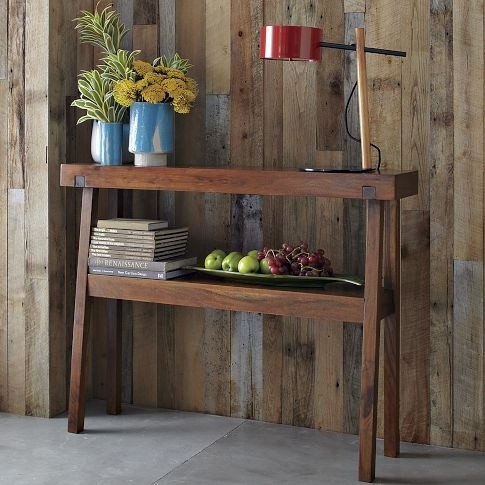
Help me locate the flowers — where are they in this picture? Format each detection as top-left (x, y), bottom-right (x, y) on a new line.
top-left (112, 58), bottom-right (198, 113)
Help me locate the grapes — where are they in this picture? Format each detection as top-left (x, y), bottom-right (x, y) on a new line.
top-left (256, 241), bottom-right (334, 277)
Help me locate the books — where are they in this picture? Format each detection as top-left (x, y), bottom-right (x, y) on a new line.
top-left (89, 218), bottom-right (198, 280)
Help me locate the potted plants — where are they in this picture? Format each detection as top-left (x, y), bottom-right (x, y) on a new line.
top-left (66, 0), bottom-right (194, 165)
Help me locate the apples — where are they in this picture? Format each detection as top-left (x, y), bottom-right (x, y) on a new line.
top-left (205, 249), bottom-right (271, 275)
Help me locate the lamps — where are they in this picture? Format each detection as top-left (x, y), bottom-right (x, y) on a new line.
top-left (257, 22), bottom-right (407, 174)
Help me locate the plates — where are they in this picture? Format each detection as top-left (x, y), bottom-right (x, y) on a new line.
top-left (183, 264), bottom-right (366, 294)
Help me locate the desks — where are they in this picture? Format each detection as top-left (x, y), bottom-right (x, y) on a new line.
top-left (57, 159), bottom-right (419, 483)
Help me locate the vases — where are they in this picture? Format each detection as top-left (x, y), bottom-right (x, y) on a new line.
top-left (126, 100), bottom-right (177, 167)
